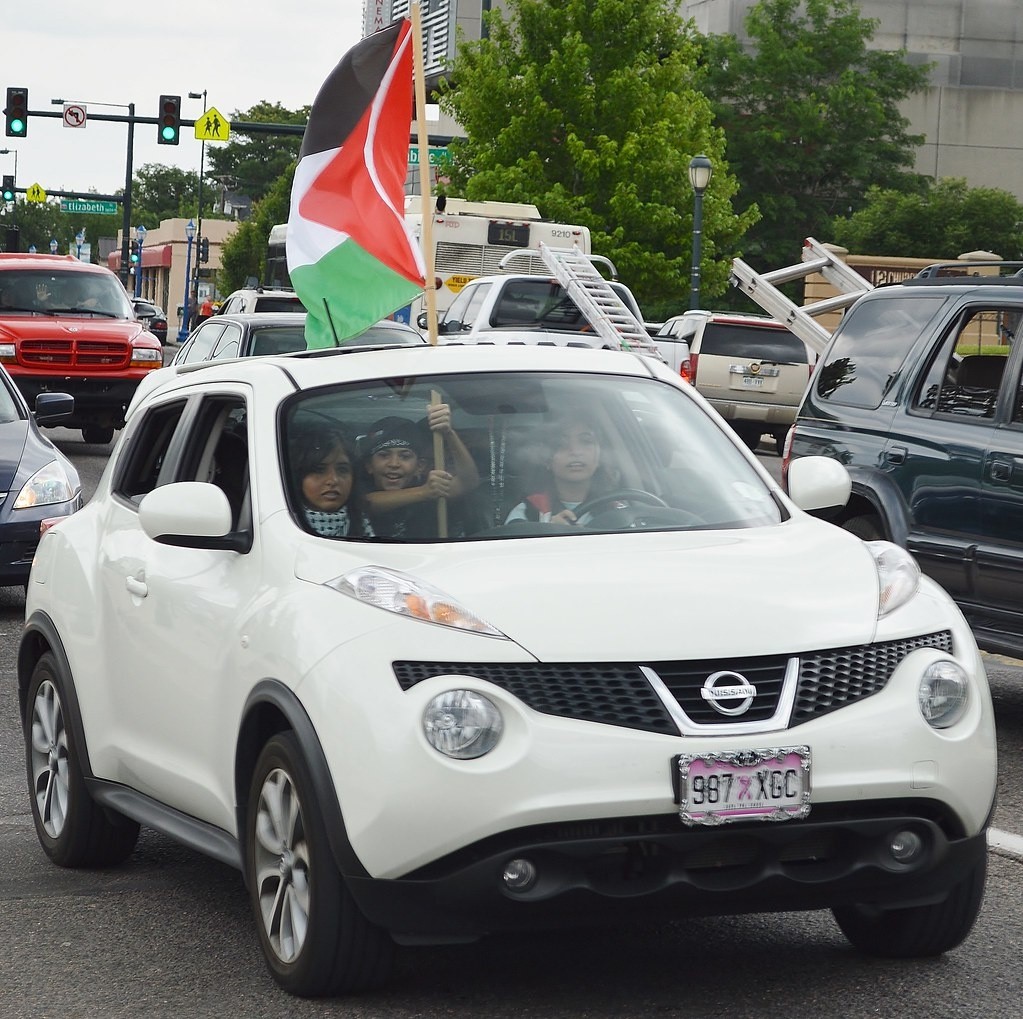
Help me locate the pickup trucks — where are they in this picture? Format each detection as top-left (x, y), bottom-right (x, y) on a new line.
top-left (416, 274), bottom-right (694, 395)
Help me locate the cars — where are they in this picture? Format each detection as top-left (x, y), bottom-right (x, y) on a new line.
top-left (0, 362), bottom-right (84, 596)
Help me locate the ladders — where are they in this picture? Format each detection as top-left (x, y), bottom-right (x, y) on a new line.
top-left (537, 239), bottom-right (669, 364)
top-left (728, 237), bottom-right (964, 392)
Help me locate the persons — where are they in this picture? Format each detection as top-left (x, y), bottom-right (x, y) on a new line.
top-left (0, 278), bottom-right (105, 310)
top-left (504, 412), bottom-right (647, 529)
top-left (284, 403), bottom-right (481, 539)
top-left (187, 290), bottom-right (221, 333)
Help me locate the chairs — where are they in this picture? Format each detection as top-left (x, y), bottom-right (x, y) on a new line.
top-left (466, 431), bottom-right (556, 531)
top-left (957, 356), bottom-right (1023, 385)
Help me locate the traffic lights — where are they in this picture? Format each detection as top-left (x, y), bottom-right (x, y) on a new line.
top-left (5, 86), bottom-right (28, 138)
top-left (156, 95), bottom-right (181, 145)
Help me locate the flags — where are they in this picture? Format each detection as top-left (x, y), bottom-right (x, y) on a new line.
top-left (285, 16), bottom-right (426, 350)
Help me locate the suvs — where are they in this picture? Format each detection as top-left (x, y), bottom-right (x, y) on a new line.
top-left (0, 247), bottom-right (164, 442)
top-left (778, 256), bottom-right (1023, 667)
top-left (163, 315), bottom-right (468, 446)
top-left (655, 308), bottom-right (820, 459)
top-left (18, 336), bottom-right (1002, 997)
top-left (211, 283), bottom-right (309, 316)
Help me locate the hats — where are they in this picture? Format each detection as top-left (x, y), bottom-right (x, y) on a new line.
top-left (363, 415), bottom-right (424, 455)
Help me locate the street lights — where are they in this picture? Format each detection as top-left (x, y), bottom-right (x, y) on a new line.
top-left (74, 233), bottom-right (84, 260)
top-left (686, 154), bottom-right (714, 308)
top-left (50, 99), bottom-right (136, 292)
top-left (136, 223), bottom-right (147, 299)
top-left (175, 219), bottom-right (197, 343)
top-left (188, 90), bottom-right (208, 329)
